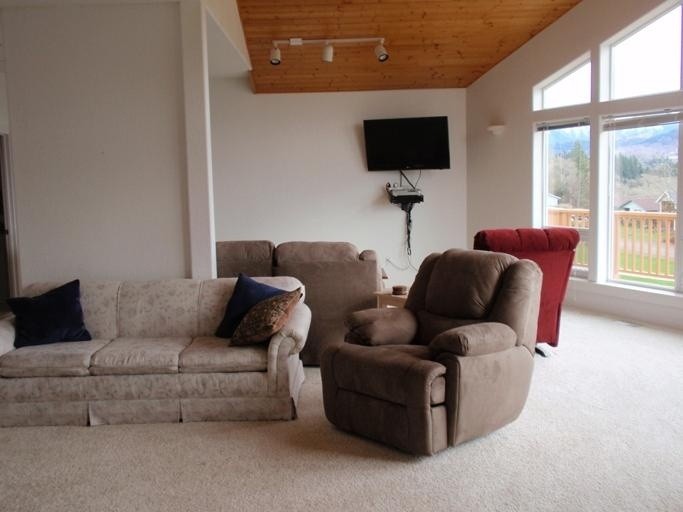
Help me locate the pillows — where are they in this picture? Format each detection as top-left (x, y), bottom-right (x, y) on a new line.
top-left (4, 279), bottom-right (91, 348)
top-left (214, 272), bottom-right (304, 348)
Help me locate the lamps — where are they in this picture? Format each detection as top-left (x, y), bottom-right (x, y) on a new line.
top-left (269, 36), bottom-right (389, 64)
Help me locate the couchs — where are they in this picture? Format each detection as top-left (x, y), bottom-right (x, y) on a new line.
top-left (216, 240), bottom-right (388, 367)
top-left (0, 274), bottom-right (313, 427)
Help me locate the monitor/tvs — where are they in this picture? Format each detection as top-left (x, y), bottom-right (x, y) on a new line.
top-left (363, 116), bottom-right (450, 172)
